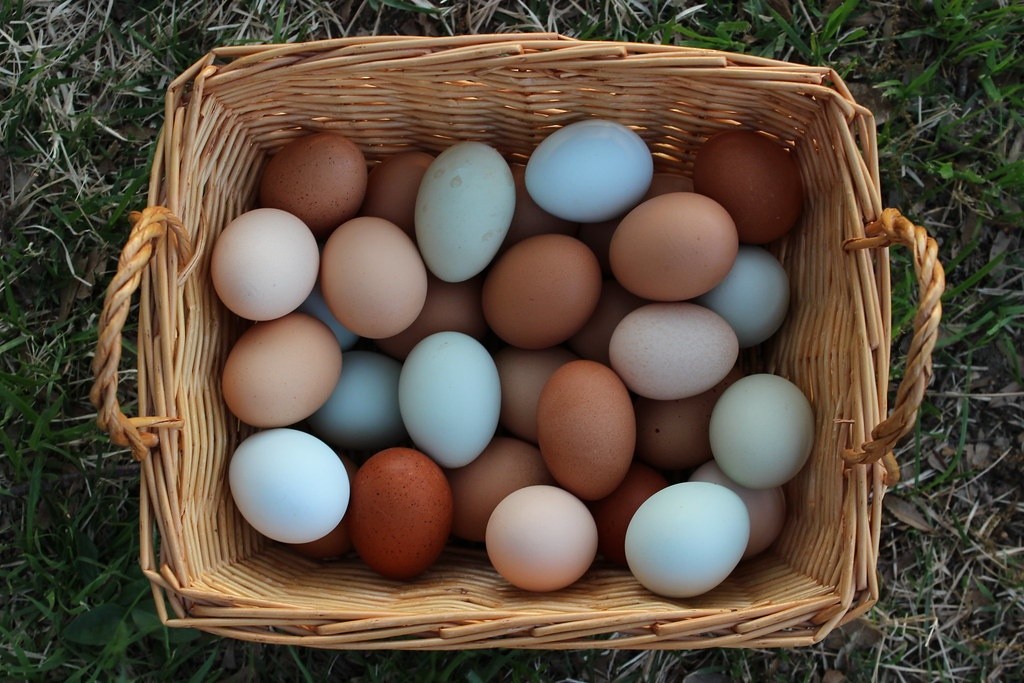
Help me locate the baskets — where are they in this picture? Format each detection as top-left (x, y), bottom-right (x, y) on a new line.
top-left (91, 31), bottom-right (946, 650)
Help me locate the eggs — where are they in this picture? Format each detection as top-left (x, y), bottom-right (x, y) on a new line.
top-left (208, 118), bottom-right (816, 599)
